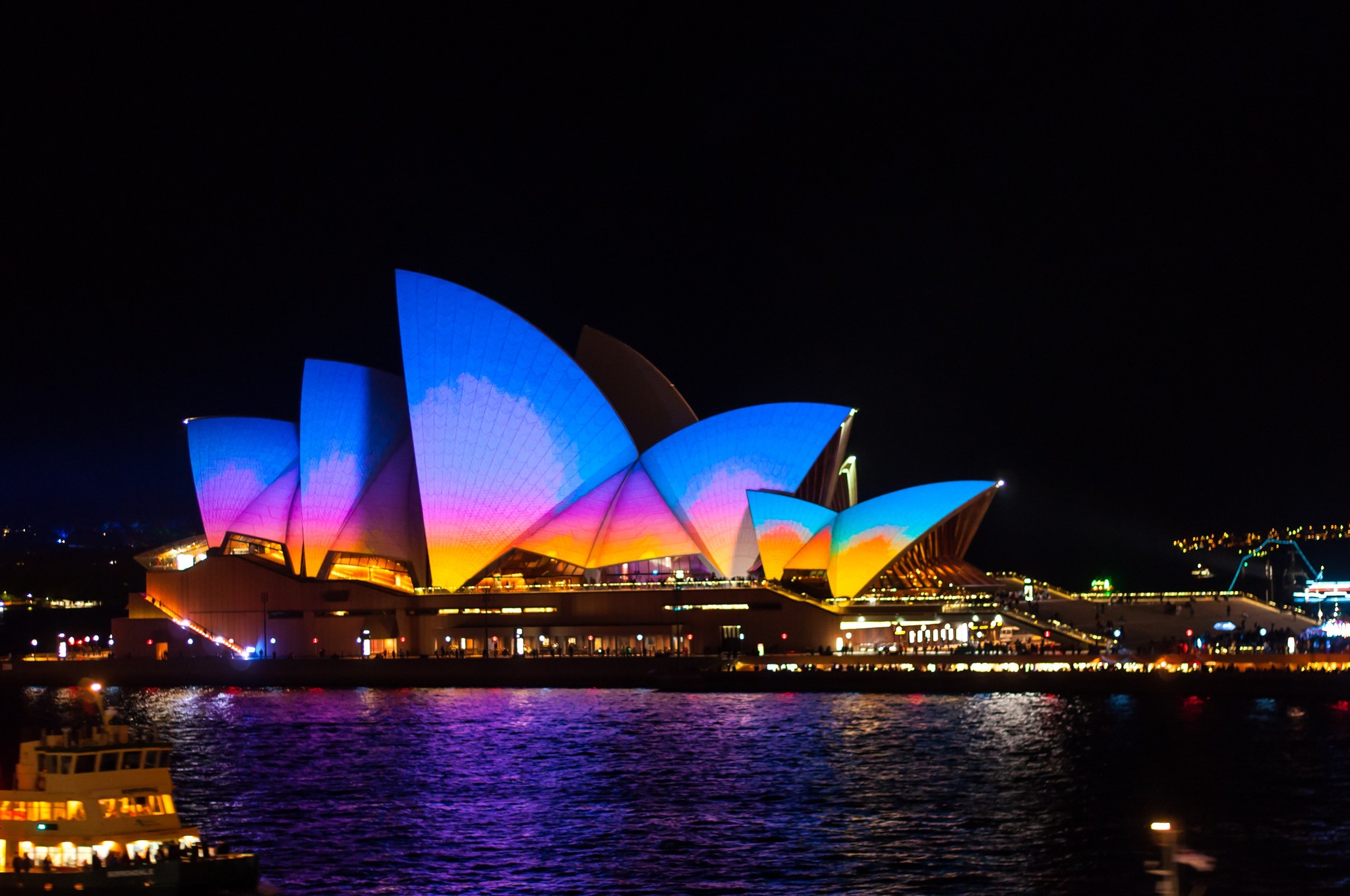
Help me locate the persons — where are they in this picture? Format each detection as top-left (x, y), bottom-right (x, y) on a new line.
top-left (11, 839), bottom-right (51, 873)
top-left (92, 839), bottom-right (182, 870)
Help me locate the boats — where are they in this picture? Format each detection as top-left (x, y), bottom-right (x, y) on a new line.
top-left (0, 684), bottom-right (258, 896)
top-left (1278, 550), bottom-right (1350, 614)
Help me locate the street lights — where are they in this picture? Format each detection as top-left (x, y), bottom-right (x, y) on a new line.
top-left (482, 588), bottom-right (491, 657)
top-left (675, 584), bottom-right (682, 657)
top-left (1187, 628), bottom-right (1193, 652)
top-left (261, 592), bottom-right (268, 658)
top-left (1114, 629), bottom-right (1121, 654)
